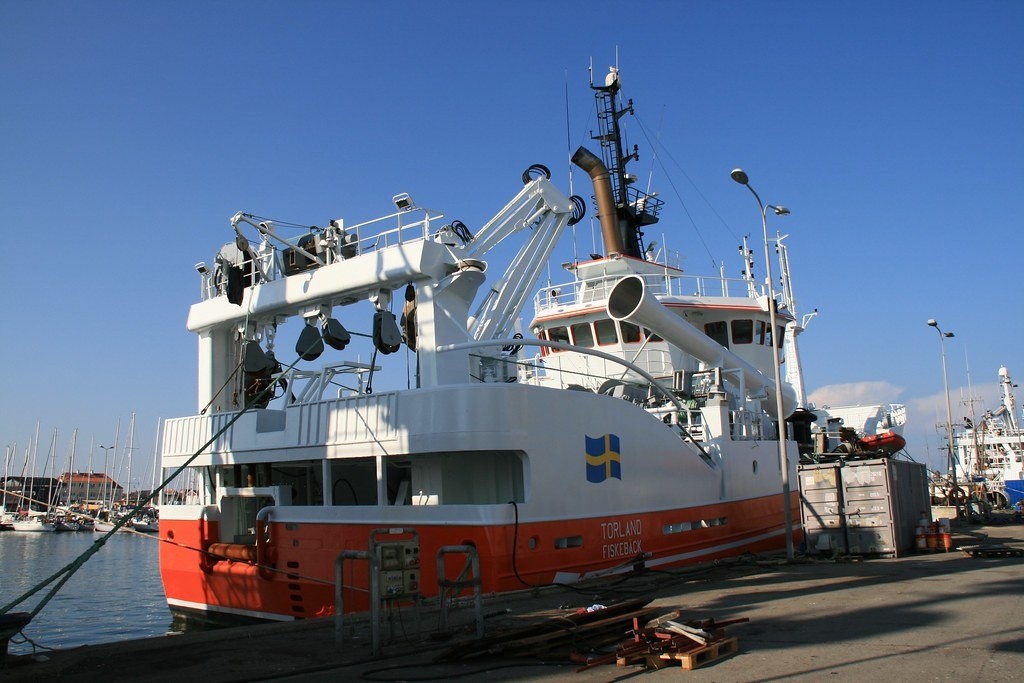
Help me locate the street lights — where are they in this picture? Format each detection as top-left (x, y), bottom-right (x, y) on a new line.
top-left (100, 445), bottom-right (114, 509)
top-left (731, 169), bottom-right (797, 562)
top-left (927, 318), bottom-right (962, 532)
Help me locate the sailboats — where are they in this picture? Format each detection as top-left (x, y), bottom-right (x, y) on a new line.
top-left (0, 411), bottom-right (157, 532)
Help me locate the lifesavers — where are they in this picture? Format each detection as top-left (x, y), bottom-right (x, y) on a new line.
top-left (947, 487), bottom-right (965, 503)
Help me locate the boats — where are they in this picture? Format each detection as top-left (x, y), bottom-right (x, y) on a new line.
top-left (157, 42), bottom-right (911, 628)
top-left (943, 343), bottom-right (1024, 508)
top-left (862, 432), bottom-right (906, 455)
